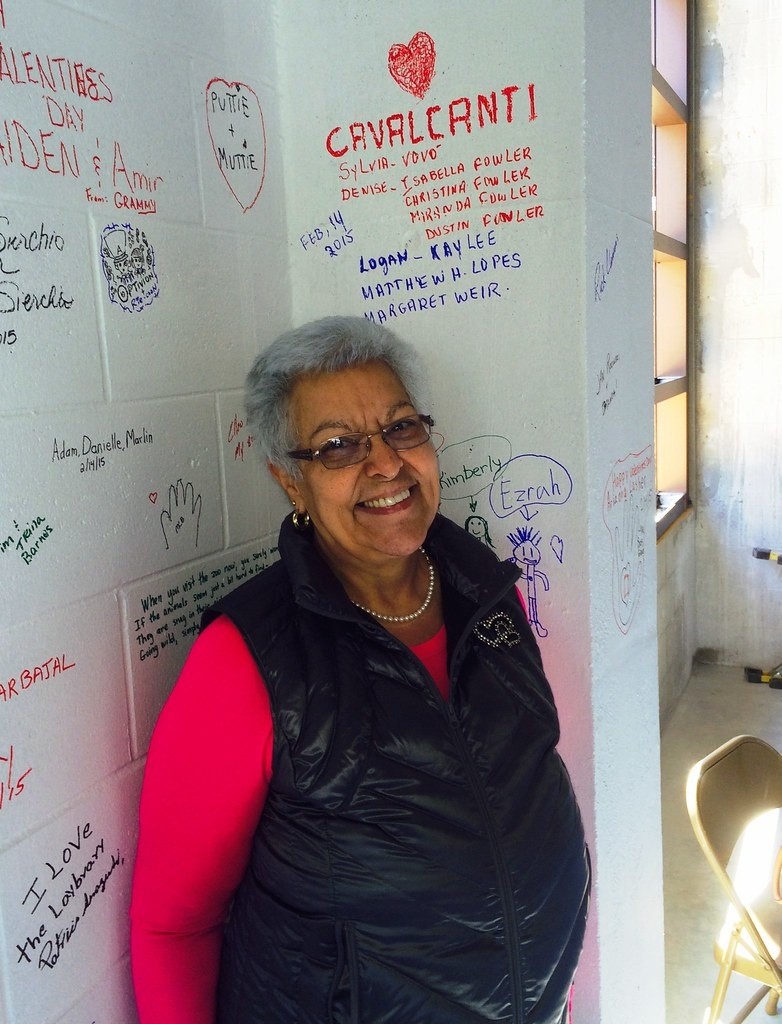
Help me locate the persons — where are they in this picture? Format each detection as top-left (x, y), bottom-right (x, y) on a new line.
top-left (128, 316), bottom-right (593, 1024)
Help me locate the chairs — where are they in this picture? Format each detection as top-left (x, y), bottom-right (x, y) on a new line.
top-left (686, 734), bottom-right (782, 1024)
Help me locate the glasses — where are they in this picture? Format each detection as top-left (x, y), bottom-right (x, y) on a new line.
top-left (286, 414), bottom-right (433, 470)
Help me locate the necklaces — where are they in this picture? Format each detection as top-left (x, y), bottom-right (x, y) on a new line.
top-left (350, 546), bottom-right (435, 623)
